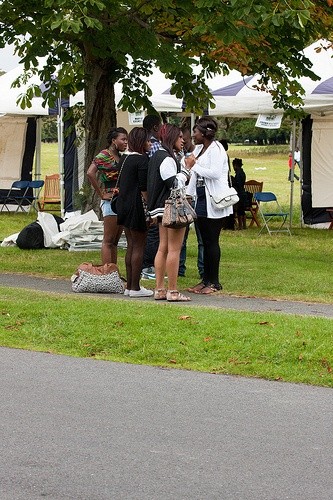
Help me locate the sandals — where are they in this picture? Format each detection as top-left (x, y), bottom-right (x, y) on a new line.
top-left (154, 288), bottom-right (167, 300)
top-left (188, 281), bottom-right (223, 294)
top-left (167, 289), bottom-right (191, 302)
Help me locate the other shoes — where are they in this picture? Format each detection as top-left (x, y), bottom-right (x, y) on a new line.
top-left (124, 286), bottom-right (153, 296)
top-left (178, 271), bottom-right (185, 276)
top-left (141, 268), bottom-right (156, 280)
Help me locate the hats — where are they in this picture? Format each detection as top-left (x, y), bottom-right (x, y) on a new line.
top-left (233, 158), bottom-right (244, 165)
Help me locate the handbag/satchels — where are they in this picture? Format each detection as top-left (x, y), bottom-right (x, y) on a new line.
top-left (210, 186), bottom-right (239, 210)
top-left (71, 262), bottom-right (124, 293)
top-left (162, 197), bottom-right (198, 230)
top-left (111, 195), bottom-right (117, 214)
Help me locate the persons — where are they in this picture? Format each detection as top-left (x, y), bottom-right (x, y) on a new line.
top-left (87, 115), bottom-right (302, 302)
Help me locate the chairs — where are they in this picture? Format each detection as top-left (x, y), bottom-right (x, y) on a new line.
top-left (0, 174), bottom-right (64, 218)
top-left (229, 179), bottom-right (292, 238)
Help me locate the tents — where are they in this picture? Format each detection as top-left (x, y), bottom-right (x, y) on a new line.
top-left (0, 38), bottom-right (333, 230)
